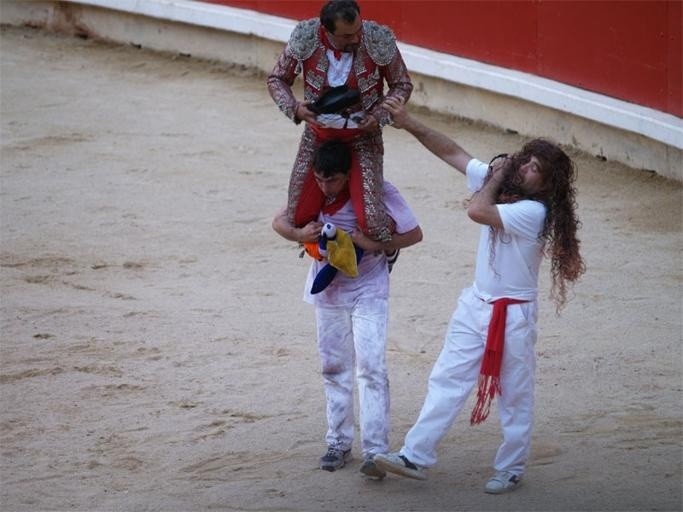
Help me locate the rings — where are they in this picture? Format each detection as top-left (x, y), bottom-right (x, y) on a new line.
top-left (312, 233), bottom-right (317, 239)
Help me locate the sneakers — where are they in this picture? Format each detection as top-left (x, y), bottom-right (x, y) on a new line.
top-left (320, 447), bottom-right (353, 472)
top-left (484, 470), bottom-right (523, 495)
top-left (359, 452), bottom-right (428, 481)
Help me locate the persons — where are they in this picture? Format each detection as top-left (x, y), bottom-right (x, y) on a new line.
top-left (267, 0), bottom-right (413, 272)
top-left (273, 138), bottom-right (424, 482)
top-left (374, 93), bottom-right (587, 495)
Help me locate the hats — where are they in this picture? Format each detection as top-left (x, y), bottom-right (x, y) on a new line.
top-left (307, 84), bottom-right (360, 114)
